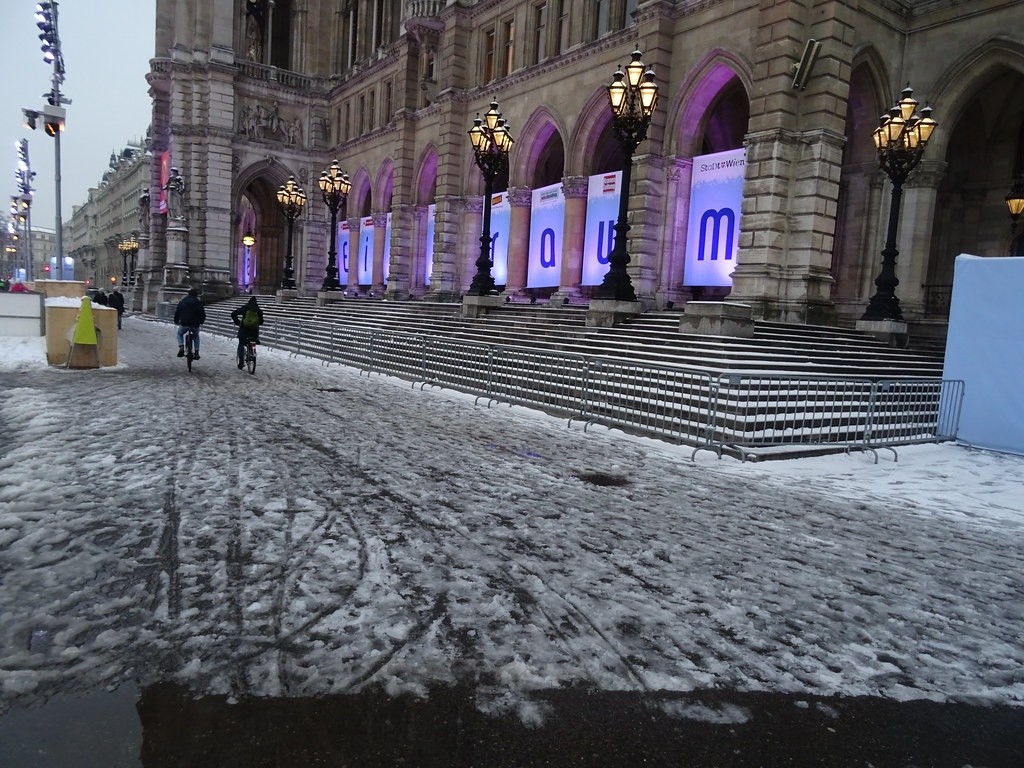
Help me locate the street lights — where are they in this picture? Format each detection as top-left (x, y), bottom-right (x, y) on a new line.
top-left (15, 137), bottom-right (37, 282)
top-left (467, 98), bottom-right (514, 296)
top-left (862, 81), bottom-right (938, 321)
top-left (596, 47), bottom-right (660, 302)
top-left (6, 194), bottom-right (21, 284)
top-left (316, 158), bottom-right (353, 292)
top-left (20, 0), bottom-right (73, 280)
top-left (118, 233), bottom-right (139, 293)
top-left (276, 173), bottom-right (308, 299)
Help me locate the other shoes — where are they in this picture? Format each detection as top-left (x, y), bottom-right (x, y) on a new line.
top-left (118, 326), bottom-right (122, 329)
top-left (238, 361), bottom-right (245, 370)
top-left (177, 345), bottom-right (185, 357)
top-left (194, 350), bottom-right (200, 360)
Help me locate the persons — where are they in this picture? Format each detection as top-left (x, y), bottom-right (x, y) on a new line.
top-left (231, 296), bottom-right (263, 369)
top-left (11, 279), bottom-right (29, 292)
top-left (109, 287), bottom-right (124, 329)
top-left (163, 167), bottom-right (185, 219)
top-left (92, 288), bottom-right (107, 306)
top-left (136, 188), bottom-right (150, 233)
top-left (0, 278), bottom-right (11, 291)
top-left (174, 289), bottom-right (206, 359)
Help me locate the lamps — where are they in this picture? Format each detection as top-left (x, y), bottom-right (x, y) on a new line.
top-left (790, 39), bottom-right (823, 92)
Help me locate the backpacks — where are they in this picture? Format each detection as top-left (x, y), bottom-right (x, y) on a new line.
top-left (244, 305), bottom-right (259, 326)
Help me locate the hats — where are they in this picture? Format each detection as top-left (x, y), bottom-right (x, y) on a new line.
top-left (189, 288), bottom-right (198, 297)
top-left (112, 287), bottom-right (118, 291)
top-left (248, 296), bottom-right (257, 302)
top-left (98, 288), bottom-right (103, 293)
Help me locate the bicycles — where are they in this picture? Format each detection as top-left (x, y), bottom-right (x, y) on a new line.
top-left (228, 322), bottom-right (258, 374)
top-left (179, 330), bottom-right (200, 372)
